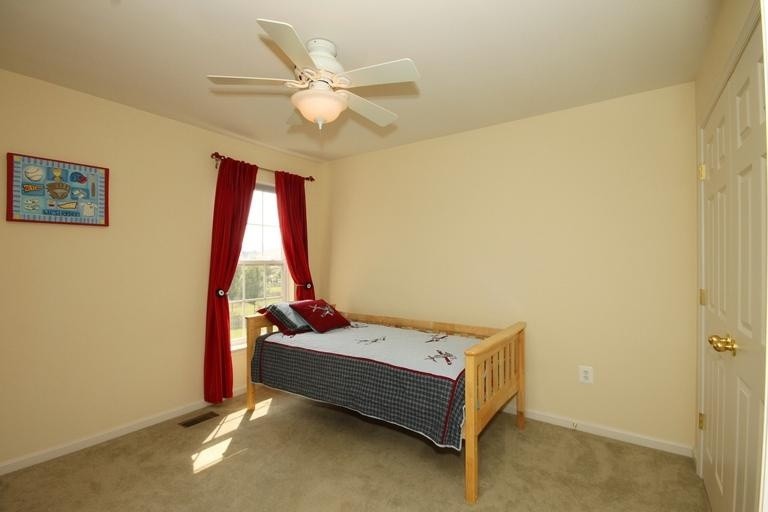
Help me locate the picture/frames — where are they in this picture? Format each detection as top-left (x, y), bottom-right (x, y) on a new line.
top-left (5, 151), bottom-right (110, 226)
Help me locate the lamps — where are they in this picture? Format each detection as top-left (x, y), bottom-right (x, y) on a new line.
top-left (285, 70), bottom-right (351, 129)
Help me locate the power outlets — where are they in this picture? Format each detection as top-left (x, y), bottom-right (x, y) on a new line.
top-left (579, 367), bottom-right (593, 384)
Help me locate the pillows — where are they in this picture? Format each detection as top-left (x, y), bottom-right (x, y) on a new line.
top-left (258, 303), bottom-right (311, 336)
top-left (289, 299), bottom-right (350, 333)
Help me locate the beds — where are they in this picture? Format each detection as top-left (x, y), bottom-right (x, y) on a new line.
top-left (245, 304), bottom-right (527, 504)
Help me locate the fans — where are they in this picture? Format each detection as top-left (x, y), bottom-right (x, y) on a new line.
top-left (206, 18), bottom-right (420, 127)
